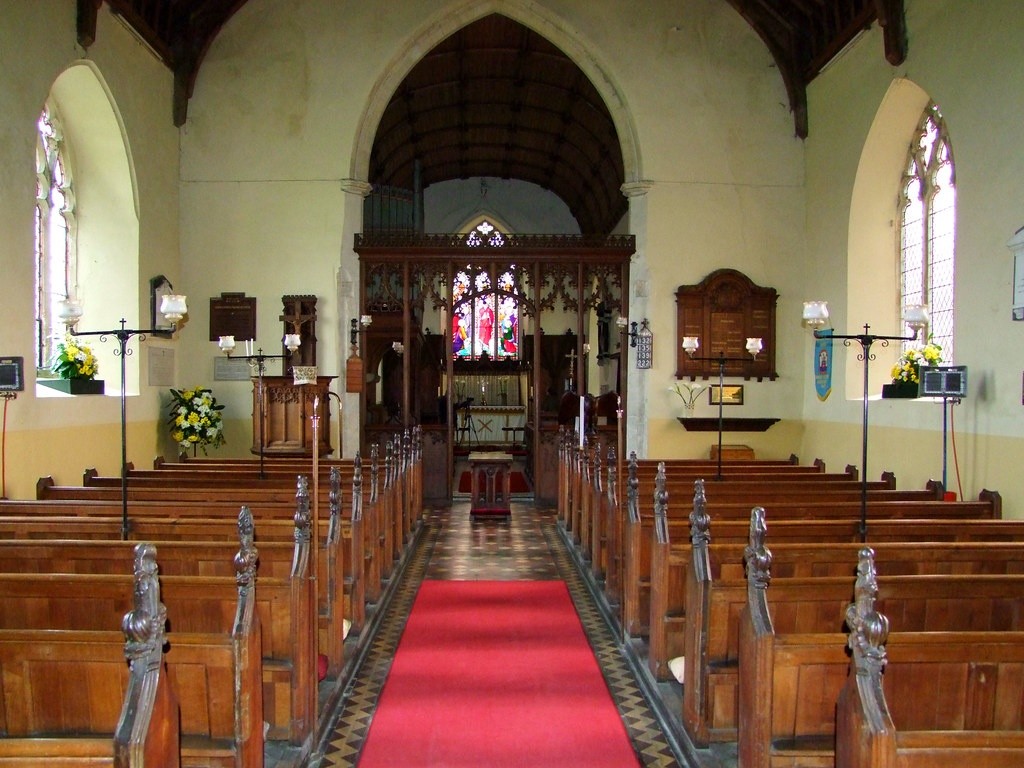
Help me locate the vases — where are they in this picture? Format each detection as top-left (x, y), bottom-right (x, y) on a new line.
top-left (684, 403), bottom-right (695, 417)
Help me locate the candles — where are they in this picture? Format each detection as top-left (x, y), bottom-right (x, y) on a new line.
top-left (251, 338), bottom-right (253, 355)
top-left (245, 340), bottom-right (249, 356)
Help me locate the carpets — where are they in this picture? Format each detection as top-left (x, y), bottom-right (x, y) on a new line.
top-left (459, 471), bottom-right (530, 493)
top-left (357, 579), bottom-right (643, 768)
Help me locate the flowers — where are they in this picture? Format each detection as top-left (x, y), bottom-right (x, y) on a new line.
top-left (50, 335), bottom-right (99, 383)
top-left (163, 385), bottom-right (227, 457)
top-left (891, 333), bottom-right (942, 384)
top-left (668, 382), bottom-right (712, 410)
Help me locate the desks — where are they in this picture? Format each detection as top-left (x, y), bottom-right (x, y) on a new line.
top-left (455, 406), bottom-right (525, 442)
top-left (468, 453), bottom-right (513, 521)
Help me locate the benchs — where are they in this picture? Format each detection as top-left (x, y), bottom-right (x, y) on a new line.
top-left (555, 426), bottom-right (1024, 768)
top-left (1, 425), bottom-right (425, 768)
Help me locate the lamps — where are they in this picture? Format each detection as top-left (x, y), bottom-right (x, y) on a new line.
top-left (351, 314), bottom-right (373, 350)
top-left (56, 295), bottom-right (187, 544)
top-left (616, 316), bottom-right (638, 347)
top-left (219, 333), bottom-right (301, 479)
top-left (682, 335), bottom-right (762, 482)
top-left (803, 301), bottom-right (932, 543)
